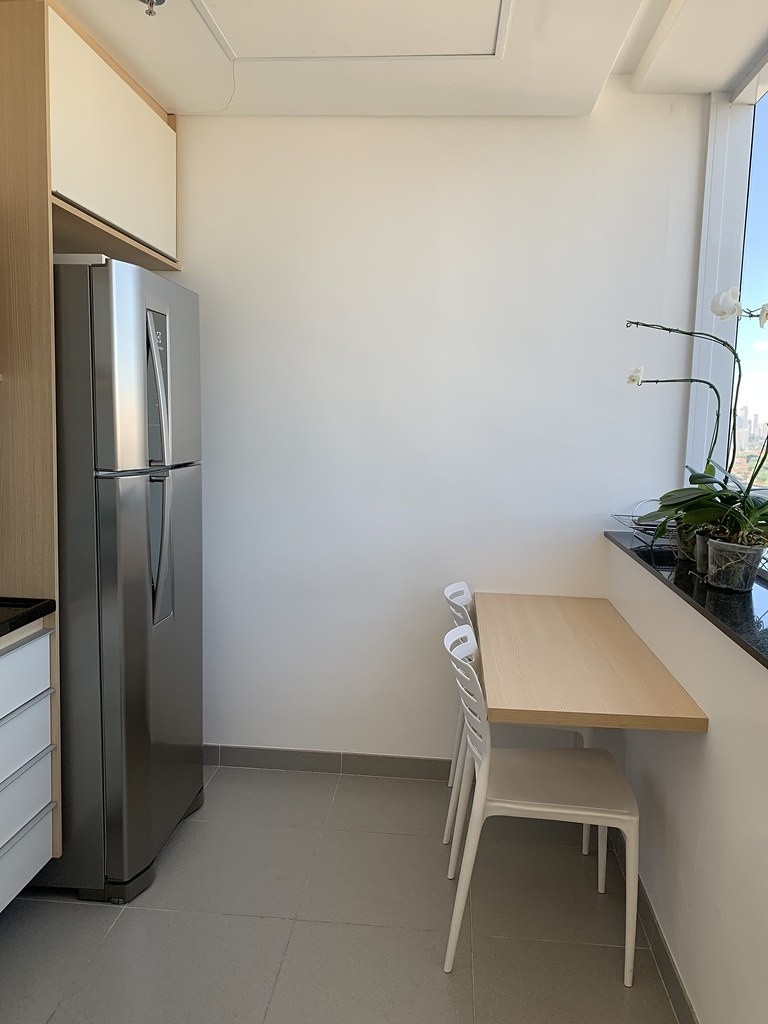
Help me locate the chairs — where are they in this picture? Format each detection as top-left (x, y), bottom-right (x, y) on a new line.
top-left (437, 580), bottom-right (645, 991)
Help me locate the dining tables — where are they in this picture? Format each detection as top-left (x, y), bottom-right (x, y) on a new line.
top-left (472, 588), bottom-right (713, 737)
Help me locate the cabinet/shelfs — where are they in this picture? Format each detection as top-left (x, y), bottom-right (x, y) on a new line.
top-left (0, 594), bottom-right (67, 922)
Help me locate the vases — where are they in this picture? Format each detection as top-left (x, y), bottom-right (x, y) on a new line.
top-left (667, 515), bottom-right (768, 592)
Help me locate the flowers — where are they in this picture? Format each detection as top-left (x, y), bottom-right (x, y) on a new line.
top-left (622, 278), bottom-right (768, 553)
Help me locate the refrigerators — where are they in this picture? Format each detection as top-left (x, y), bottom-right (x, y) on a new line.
top-left (23, 249), bottom-right (206, 903)
